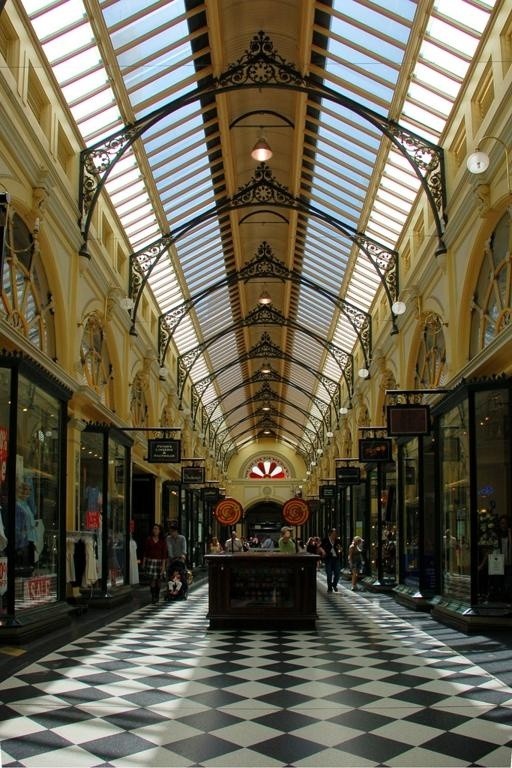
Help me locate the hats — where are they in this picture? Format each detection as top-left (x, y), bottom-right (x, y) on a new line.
top-left (281, 526), bottom-right (293, 531)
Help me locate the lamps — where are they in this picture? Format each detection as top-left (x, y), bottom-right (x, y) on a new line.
top-left (302, 287), bottom-right (420, 482)
top-left (461, 133), bottom-right (508, 175)
top-left (183, 408), bottom-right (232, 483)
top-left (258, 279), bottom-right (271, 435)
top-left (158, 366), bottom-right (169, 379)
top-left (118, 294), bottom-right (134, 310)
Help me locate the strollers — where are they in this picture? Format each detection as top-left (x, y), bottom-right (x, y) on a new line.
top-left (163, 556), bottom-right (188, 601)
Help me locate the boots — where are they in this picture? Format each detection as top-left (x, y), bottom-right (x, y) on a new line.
top-left (151, 586), bottom-right (161, 603)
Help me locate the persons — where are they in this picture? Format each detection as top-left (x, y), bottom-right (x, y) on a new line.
top-left (347, 535), bottom-right (365, 591)
top-left (320, 528), bottom-right (345, 593)
top-left (17, 475), bottom-right (46, 567)
top-left (140, 523), bottom-right (169, 604)
top-left (166, 524), bottom-right (190, 571)
top-left (166, 569), bottom-right (183, 596)
top-left (313, 536), bottom-right (325, 571)
top-left (208, 527), bottom-right (323, 554)
top-left (443, 529), bottom-right (458, 576)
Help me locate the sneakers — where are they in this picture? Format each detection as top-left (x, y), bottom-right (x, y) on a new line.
top-left (350, 586), bottom-right (361, 591)
top-left (327, 588), bottom-right (332, 592)
top-left (334, 586), bottom-right (337, 591)
